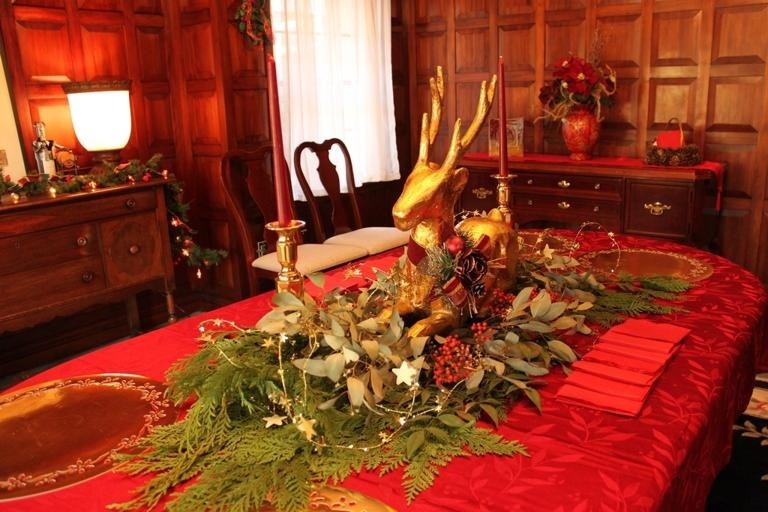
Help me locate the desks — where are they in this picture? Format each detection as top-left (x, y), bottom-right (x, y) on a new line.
top-left (0, 226), bottom-right (767, 512)
top-left (0, 177), bottom-right (176, 338)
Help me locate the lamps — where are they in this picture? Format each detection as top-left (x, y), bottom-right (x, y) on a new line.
top-left (60, 77), bottom-right (132, 152)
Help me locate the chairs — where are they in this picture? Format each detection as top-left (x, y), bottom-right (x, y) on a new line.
top-left (294, 137), bottom-right (421, 255)
top-left (217, 134), bottom-right (370, 297)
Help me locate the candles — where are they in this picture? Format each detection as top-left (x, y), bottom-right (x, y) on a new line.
top-left (497, 54), bottom-right (508, 175)
top-left (267, 56), bottom-right (294, 229)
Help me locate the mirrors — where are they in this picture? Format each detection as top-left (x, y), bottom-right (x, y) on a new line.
top-left (0, 33), bottom-right (32, 189)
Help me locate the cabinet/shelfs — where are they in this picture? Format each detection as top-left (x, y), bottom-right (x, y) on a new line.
top-left (452, 167), bottom-right (728, 258)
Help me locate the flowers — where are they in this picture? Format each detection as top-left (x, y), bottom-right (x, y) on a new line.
top-left (534, 51), bottom-right (618, 117)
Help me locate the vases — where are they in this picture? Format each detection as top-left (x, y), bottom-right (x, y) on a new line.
top-left (559, 103), bottom-right (599, 159)
